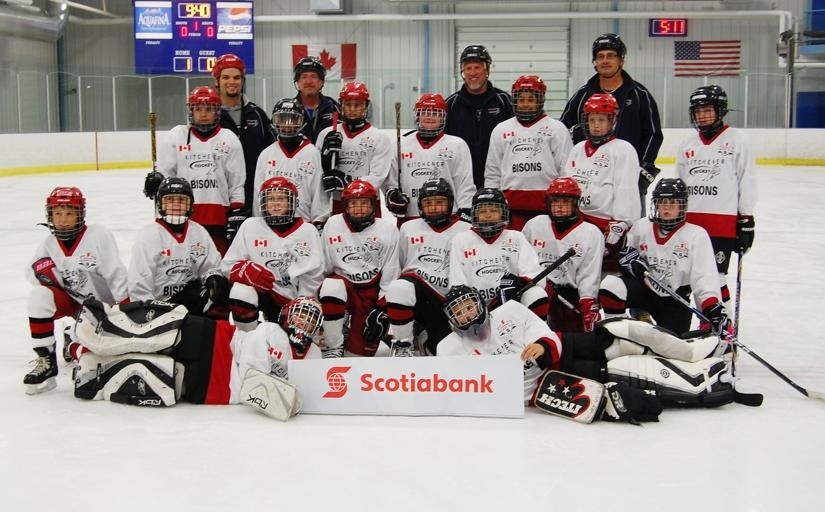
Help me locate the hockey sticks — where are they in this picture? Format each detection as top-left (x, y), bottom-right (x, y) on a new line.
top-left (730, 247), bottom-right (742, 389)
top-left (645, 270), bottom-right (824, 402)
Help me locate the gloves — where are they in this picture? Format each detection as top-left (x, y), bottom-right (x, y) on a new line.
top-left (205, 275), bottom-right (228, 304)
top-left (142, 171), bottom-right (164, 195)
top-left (579, 298), bottom-right (602, 333)
top-left (361, 308), bottom-right (390, 343)
top-left (698, 301), bottom-right (727, 339)
top-left (230, 257), bottom-right (274, 294)
top-left (732, 219), bottom-right (754, 255)
top-left (318, 132), bottom-right (343, 156)
top-left (385, 188), bottom-right (411, 219)
top-left (499, 272), bottom-right (525, 305)
top-left (31, 257), bottom-right (64, 289)
top-left (322, 169), bottom-right (352, 192)
top-left (617, 248), bottom-right (649, 280)
top-left (457, 208), bottom-right (472, 223)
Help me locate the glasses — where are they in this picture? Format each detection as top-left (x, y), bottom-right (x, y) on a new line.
top-left (595, 53), bottom-right (618, 61)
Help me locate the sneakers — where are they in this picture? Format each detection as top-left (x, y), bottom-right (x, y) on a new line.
top-left (391, 339), bottom-right (414, 358)
top-left (22, 342), bottom-right (59, 384)
top-left (698, 320), bottom-right (735, 342)
top-left (62, 327), bottom-right (74, 363)
top-left (629, 309), bottom-right (655, 326)
top-left (323, 334), bottom-right (346, 357)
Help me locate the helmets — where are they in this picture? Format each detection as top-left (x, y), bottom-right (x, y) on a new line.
top-left (470, 188), bottom-right (509, 236)
top-left (689, 85), bottom-right (728, 132)
top-left (511, 75), bottom-right (547, 125)
top-left (545, 177), bottom-right (583, 227)
top-left (647, 178), bottom-right (688, 231)
top-left (187, 53), bottom-right (370, 138)
top-left (593, 33), bottom-right (627, 79)
top-left (442, 285), bottom-right (487, 335)
top-left (44, 186), bottom-right (87, 241)
top-left (414, 94), bottom-right (447, 143)
top-left (460, 45), bottom-right (491, 64)
top-left (341, 180), bottom-right (378, 226)
top-left (259, 177), bottom-right (299, 225)
top-left (280, 297), bottom-right (325, 354)
top-left (153, 176), bottom-right (195, 227)
top-left (582, 95), bottom-right (620, 144)
top-left (416, 177), bottom-right (454, 225)
